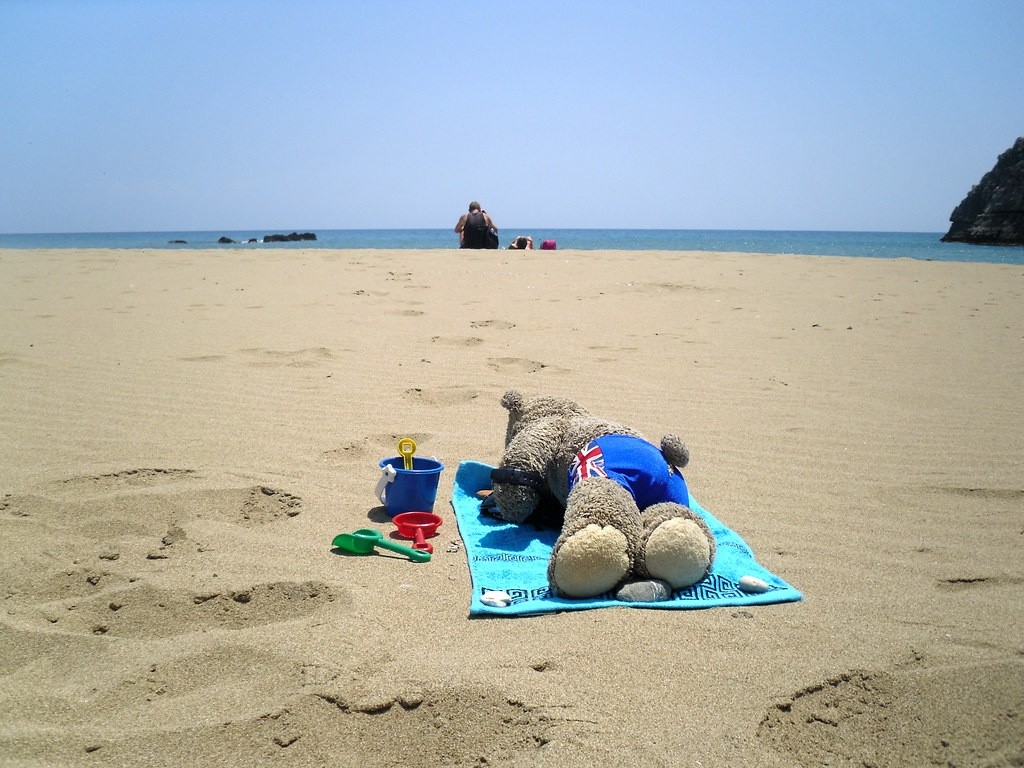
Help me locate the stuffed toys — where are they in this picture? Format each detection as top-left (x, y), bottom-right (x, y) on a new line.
top-left (476, 389), bottom-right (715, 600)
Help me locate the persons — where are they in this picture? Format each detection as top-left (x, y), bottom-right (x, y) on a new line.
top-left (454, 202), bottom-right (498, 249)
top-left (509, 237), bottom-right (532, 249)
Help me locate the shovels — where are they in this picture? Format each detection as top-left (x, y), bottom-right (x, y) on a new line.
top-left (330, 525), bottom-right (430, 563)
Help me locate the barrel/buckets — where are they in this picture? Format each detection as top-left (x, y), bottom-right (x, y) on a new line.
top-left (374, 456), bottom-right (445, 519)
